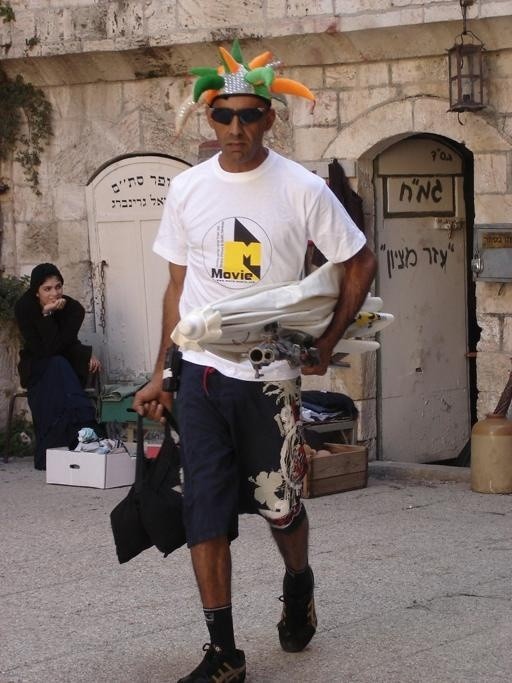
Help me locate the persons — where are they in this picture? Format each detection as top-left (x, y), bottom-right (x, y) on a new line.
top-left (126, 94), bottom-right (379, 683)
top-left (12, 262), bottom-right (103, 472)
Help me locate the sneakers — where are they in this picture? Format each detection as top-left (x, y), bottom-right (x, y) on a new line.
top-left (175, 642), bottom-right (246, 682)
top-left (276, 568), bottom-right (318, 652)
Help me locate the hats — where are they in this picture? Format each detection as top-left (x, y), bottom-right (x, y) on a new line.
top-left (171, 37), bottom-right (315, 144)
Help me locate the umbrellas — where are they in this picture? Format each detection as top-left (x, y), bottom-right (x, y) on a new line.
top-left (167, 253), bottom-right (396, 362)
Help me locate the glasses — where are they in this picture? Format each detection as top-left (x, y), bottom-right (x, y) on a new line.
top-left (205, 102), bottom-right (269, 126)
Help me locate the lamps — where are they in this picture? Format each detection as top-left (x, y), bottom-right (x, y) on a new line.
top-left (446, 0), bottom-right (486, 114)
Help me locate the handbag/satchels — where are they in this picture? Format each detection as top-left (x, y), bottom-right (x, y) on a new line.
top-left (110, 435), bottom-right (185, 565)
